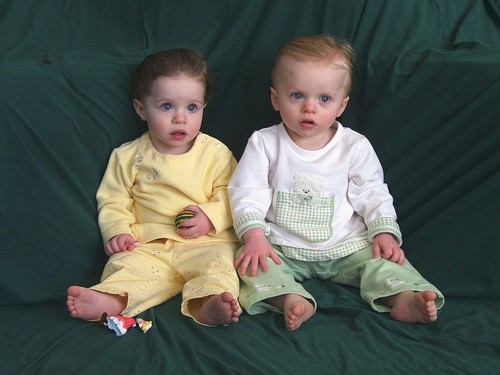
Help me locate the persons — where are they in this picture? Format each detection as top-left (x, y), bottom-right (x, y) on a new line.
top-left (100, 312), bottom-right (142, 336)
top-left (67, 46), bottom-right (242, 327)
top-left (228, 35), bottom-right (444, 330)
